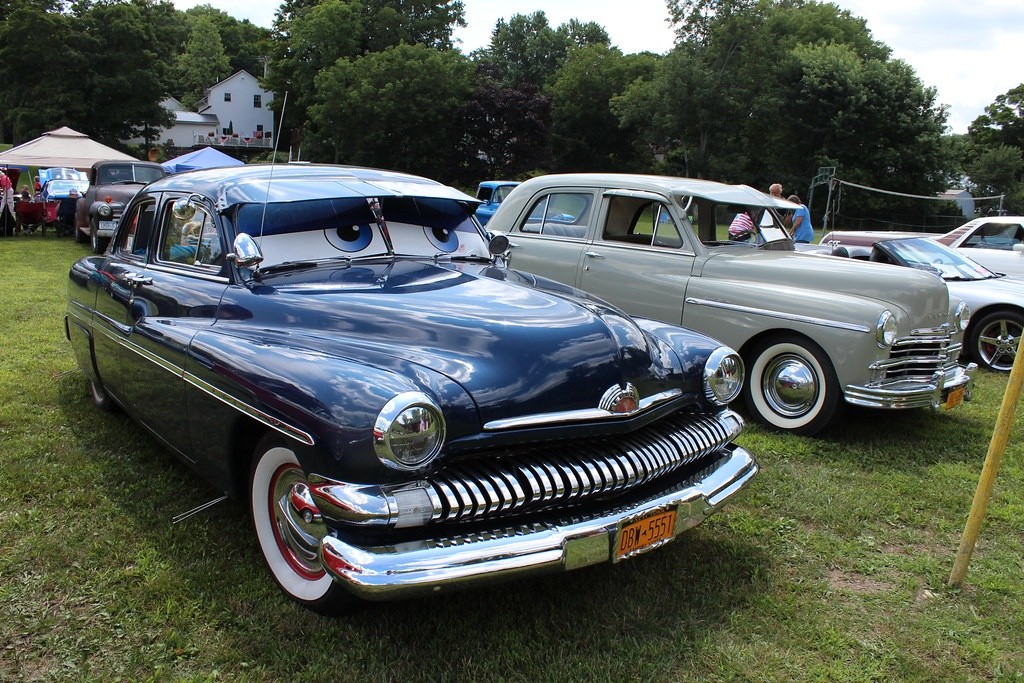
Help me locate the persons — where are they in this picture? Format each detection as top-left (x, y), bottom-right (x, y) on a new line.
top-left (0, 171), bottom-right (80, 237)
top-left (769, 183), bottom-right (792, 227)
top-left (784, 195), bottom-right (814, 243)
top-left (728, 210), bottom-right (757, 241)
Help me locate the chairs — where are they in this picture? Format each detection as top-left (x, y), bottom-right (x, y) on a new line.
top-left (15, 201), bottom-right (45, 235)
top-left (869, 247), bottom-right (889, 264)
top-left (54, 198), bottom-right (77, 237)
top-left (162, 138), bottom-right (173, 146)
top-left (831, 246), bottom-right (849, 258)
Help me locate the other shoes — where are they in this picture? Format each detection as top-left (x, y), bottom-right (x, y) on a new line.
top-left (29, 231), bottom-right (33, 236)
top-left (22, 230), bottom-right (28, 236)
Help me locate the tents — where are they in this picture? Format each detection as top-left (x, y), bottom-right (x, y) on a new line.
top-left (161, 146), bottom-right (246, 174)
top-left (0, 126), bottom-right (140, 169)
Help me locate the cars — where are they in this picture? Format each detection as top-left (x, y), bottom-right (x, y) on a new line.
top-left (798, 234), bottom-right (1024, 373)
top-left (819, 215), bottom-right (1024, 278)
top-left (64, 164), bottom-right (759, 609)
top-left (473, 180), bottom-right (575, 228)
top-left (482, 173), bottom-right (980, 438)
top-left (75, 160), bottom-right (166, 253)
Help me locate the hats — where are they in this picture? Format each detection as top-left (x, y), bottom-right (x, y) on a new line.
top-left (70, 188), bottom-right (78, 194)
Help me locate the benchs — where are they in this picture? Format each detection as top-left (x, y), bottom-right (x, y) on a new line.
top-left (541, 223), bottom-right (588, 238)
top-left (602, 233), bottom-right (654, 245)
